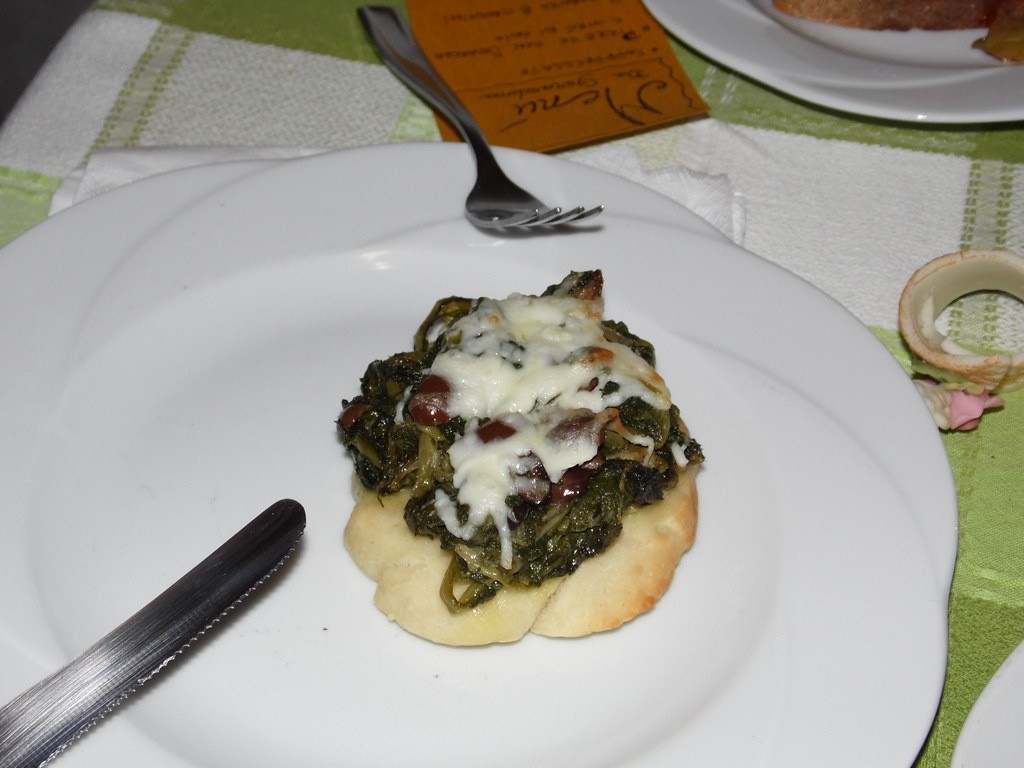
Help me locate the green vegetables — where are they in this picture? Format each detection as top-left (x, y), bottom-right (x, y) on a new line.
top-left (334, 266), bottom-right (702, 612)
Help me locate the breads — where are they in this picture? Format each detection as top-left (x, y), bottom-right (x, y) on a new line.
top-left (346, 417), bottom-right (701, 646)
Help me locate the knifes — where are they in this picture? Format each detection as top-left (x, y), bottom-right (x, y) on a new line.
top-left (5, 497), bottom-right (305, 767)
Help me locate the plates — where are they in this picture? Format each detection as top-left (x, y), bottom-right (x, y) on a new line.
top-left (954, 634), bottom-right (1023, 765)
top-left (3, 143), bottom-right (956, 767)
top-left (643, 0), bottom-right (1023, 123)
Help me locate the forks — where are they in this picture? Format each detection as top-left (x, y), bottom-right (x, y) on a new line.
top-left (359, 5), bottom-right (604, 229)
